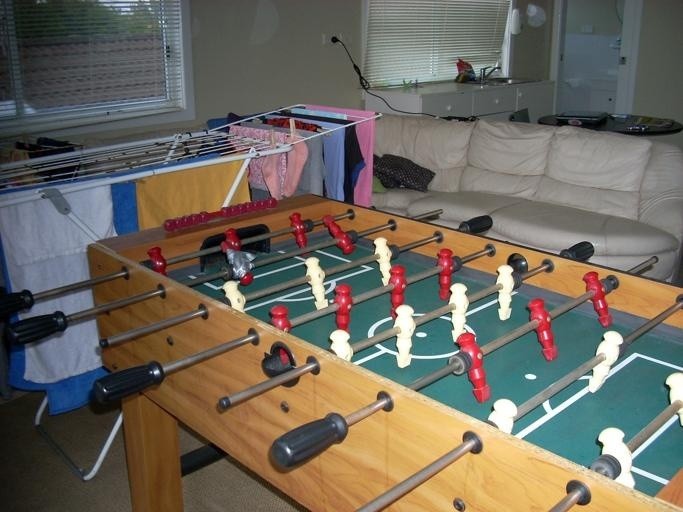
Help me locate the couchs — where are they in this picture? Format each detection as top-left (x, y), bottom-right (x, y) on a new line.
top-left (372, 112), bottom-right (683, 282)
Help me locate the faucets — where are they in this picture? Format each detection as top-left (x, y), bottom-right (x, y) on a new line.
top-left (484, 66), bottom-right (502, 82)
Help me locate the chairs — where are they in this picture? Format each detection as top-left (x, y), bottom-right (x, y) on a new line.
top-left (510, 109), bottom-right (530, 123)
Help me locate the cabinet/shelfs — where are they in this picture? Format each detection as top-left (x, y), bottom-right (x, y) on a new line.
top-left (0, 104), bottom-right (383, 480)
top-left (365, 84), bottom-right (554, 128)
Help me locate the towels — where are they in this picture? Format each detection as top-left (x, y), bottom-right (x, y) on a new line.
top-left (135, 153), bottom-right (250, 228)
top-left (3, 154), bottom-right (223, 414)
top-left (2, 180), bottom-right (118, 387)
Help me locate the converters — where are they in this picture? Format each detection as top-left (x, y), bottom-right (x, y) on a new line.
top-left (445, 116), bottom-right (466, 121)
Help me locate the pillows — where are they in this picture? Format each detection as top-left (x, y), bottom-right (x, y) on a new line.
top-left (373, 154), bottom-right (435, 192)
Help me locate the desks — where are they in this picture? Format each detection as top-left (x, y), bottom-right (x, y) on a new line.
top-left (537, 113), bottom-right (682, 136)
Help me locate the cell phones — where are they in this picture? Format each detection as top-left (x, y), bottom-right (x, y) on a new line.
top-left (627, 124), bottom-right (648, 130)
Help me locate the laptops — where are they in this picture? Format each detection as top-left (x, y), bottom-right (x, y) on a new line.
top-left (556, 111), bottom-right (608, 126)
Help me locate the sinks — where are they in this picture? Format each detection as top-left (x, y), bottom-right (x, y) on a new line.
top-left (464, 76), bottom-right (543, 86)
